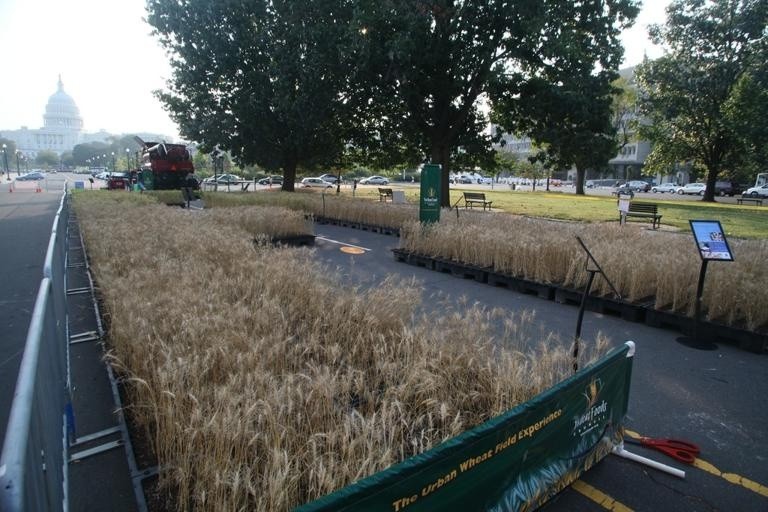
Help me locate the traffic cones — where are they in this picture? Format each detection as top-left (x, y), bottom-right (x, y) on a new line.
top-left (36, 185), bottom-right (43, 193)
top-left (8, 183), bottom-right (16, 193)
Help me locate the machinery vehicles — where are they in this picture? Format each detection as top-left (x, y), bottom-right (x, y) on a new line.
top-left (130, 142), bottom-right (199, 190)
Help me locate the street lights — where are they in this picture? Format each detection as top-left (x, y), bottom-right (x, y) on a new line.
top-left (103, 153), bottom-right (106, 172)
top-left (126, 148), bottom-right (130, 173)
top-left (0, 144), bottom-right (11, 180)
top-left (15, 148), bottom-right (20, 176)
top-left (112, 151), bottom-right (115, 172)
top-left (92, 156), bottom-right (95, 172)
top-left (20, 154), bottom-right (24, 175)
top-left (88, 158), bottom-right (91, 170)
top-left (25, 157), bottom-right (28, 173)
top-left (97, 155), bottom-right (100, 171)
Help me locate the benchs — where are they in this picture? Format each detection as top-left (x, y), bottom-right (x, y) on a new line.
top-left (378, 187), bottom-right (404, 203)
top-left (736, 193), bottom-right (765, 206)
top-left (463, 192), bottom-right (492, 211)
top-left (619, 202), bottom-right (663, 229)
top-left (611, 187), bottom-right (634, 197)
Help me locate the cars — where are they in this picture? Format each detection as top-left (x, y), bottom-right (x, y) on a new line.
top-left (360, 175), bottom-right (390, 185)
top-left (106, 171), bottom-right (137, 189)
top-left (259, 175), bottom-right (284, 185)
top-left (318, 174), bottom-right (343, 185)
top-left (92, 171), bottom-right (117, 180)
top-left (16, 169), bottom-right (45, 181)
top-left (50, 169), bottom-right (57, 174)
top-left (300, 177), bottom-right (337, 188)
top-left (203, 174), bottom-right (231, 185)
top-left (207, 174), bottom-right (245, 185)
